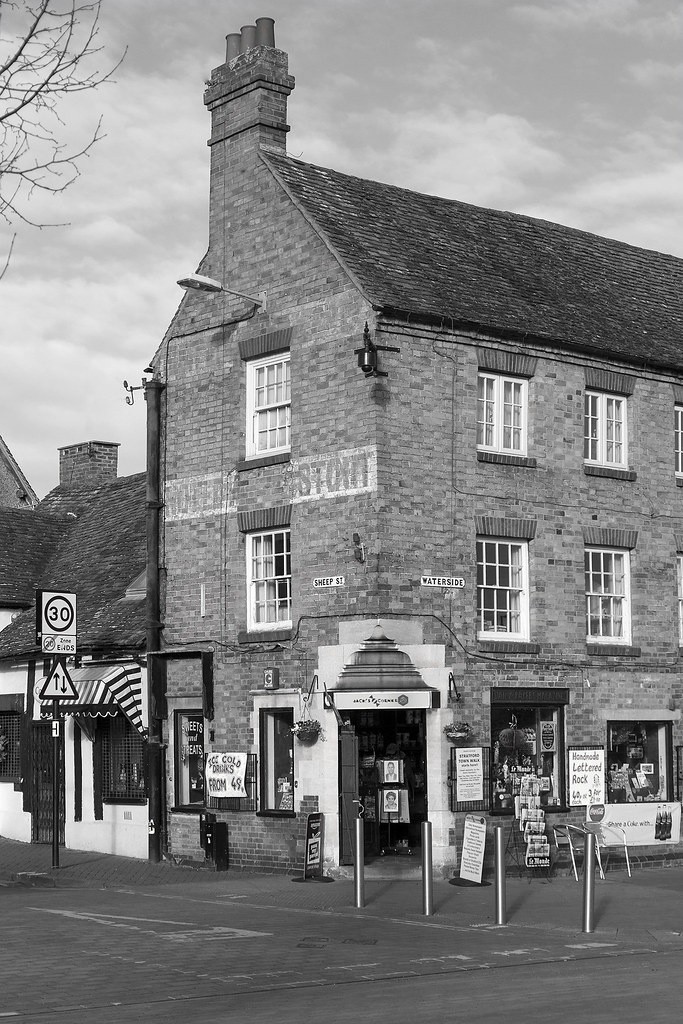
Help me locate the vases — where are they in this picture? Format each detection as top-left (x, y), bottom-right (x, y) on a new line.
top-left (297, 732), bottom-right (318, 742)
top-left (447, 732), bottom-right (470, 742)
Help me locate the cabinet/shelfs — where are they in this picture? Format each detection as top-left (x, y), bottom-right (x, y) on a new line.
top-left (506, 795), bottom-right (552, 884)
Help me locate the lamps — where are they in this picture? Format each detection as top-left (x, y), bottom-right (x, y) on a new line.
top-left (355, 321), bottom-right (401, 377)
top-left (124, 378), bottom-right (147, 405)
top-left (177, 273), bottom-right (266, 315)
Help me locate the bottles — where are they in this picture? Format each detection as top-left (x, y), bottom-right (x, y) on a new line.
top-left (656, 804), bottom-right (672, 842)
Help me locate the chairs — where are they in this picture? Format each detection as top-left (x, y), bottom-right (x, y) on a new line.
top-left (551, 821), bottom-right (632, 882)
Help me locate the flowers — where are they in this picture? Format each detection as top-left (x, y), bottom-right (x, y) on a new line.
top-left (443, 721), bottom-right (473, 736)
top-left (291, 720), bottom-right (325, 742)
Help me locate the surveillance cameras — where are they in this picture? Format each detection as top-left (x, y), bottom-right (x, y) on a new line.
top-left (357, 348), bottom-right (374, 372)
